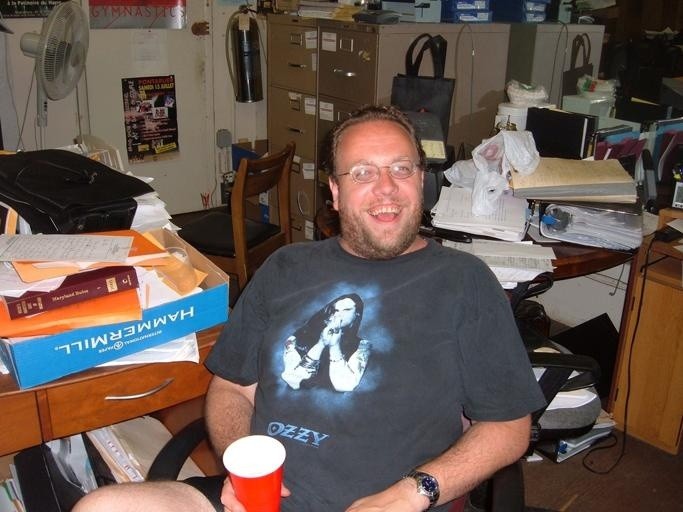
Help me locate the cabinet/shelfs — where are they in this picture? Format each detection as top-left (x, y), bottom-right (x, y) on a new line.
top-left (605, 207), bottom-right (683, 457)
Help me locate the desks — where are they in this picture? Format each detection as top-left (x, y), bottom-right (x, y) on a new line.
top-left (1, 307), bottom-right (232, 457)
top-left (314, 204), bottom-right (638, 285)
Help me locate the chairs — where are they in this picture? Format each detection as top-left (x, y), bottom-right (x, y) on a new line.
top-left (176, 139), bottom-right (296, 292)
top-left (147, 417), bottom-right (524, 512)
top-left (524, 338), bottom-right (602, 457)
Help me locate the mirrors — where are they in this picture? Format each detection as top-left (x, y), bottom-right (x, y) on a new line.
top-left (489, 0), bottom-right (683, 211)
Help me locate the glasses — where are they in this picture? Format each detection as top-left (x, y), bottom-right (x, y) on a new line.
top-left (332, 158), bottom-right (426, 186)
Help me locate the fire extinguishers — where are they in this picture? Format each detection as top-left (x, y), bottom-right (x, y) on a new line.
top-left (226, 4), bottom-right (263, 103)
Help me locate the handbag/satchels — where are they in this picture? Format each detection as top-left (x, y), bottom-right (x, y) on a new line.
top-left (0, 149), bottom-right (156, 234)
top-left (390, 73), bottom-right (455, 142)
top-left (561, 64), bottom-right (593, 109)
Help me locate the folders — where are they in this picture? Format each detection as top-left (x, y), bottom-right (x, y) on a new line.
top-left (616, 96), bottom-right (673, 125)
top-left (526, 107), bottom-right (599, 160)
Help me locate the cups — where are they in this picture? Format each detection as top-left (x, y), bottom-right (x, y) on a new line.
top-left (221, 434), bottom-right (287, 512)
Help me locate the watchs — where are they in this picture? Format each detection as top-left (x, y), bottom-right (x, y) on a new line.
top-left (403, 470), bottom-right (440, 512)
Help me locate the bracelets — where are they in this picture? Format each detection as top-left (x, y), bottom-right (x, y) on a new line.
top-left (329, 357), bottom-right (345, 362)
top-left (299, 355), bottom-right (319, 369)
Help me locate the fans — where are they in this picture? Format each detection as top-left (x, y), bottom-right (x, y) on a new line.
top-left (20, 1), bottom-right (90, 150)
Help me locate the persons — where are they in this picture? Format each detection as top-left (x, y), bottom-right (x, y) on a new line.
top-left (281, 293), bottom-right (372, 392)
top-left (71, 103), bottom-right (547, 512)
